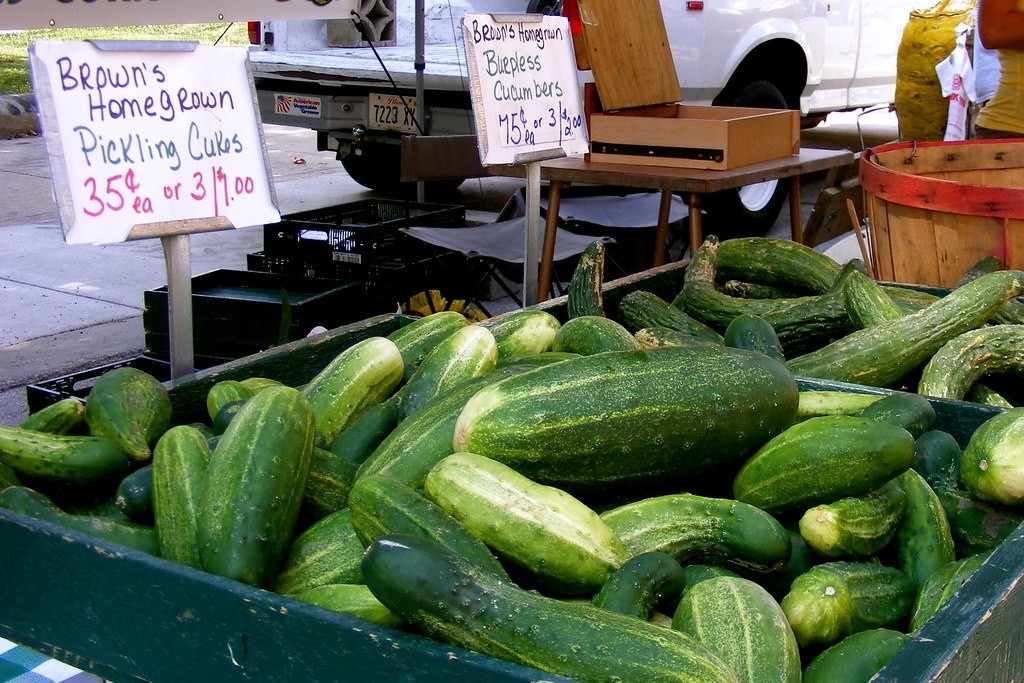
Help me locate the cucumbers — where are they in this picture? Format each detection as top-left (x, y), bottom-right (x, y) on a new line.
top-left (0, 236), bottom-right (1024, 683)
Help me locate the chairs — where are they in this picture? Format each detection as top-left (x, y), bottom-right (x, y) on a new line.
top-left (397, 132), bottom-right (616, 322)
top-left (535, 183), bottom-right (708, 280)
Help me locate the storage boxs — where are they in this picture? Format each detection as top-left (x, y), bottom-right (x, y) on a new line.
top-left (527, 259), bottom-right (957, 314)
top-left (577, 0), bottom-right (802, 172)
top-left (1, 311), bottom-right (1024, 683)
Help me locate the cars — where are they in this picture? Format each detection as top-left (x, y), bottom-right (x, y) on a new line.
top-left (234, 0), bottom-right (950, 238)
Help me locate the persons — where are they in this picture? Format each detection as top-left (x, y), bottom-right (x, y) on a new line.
top-left (975, 0), bottom-right (1024, 140)
